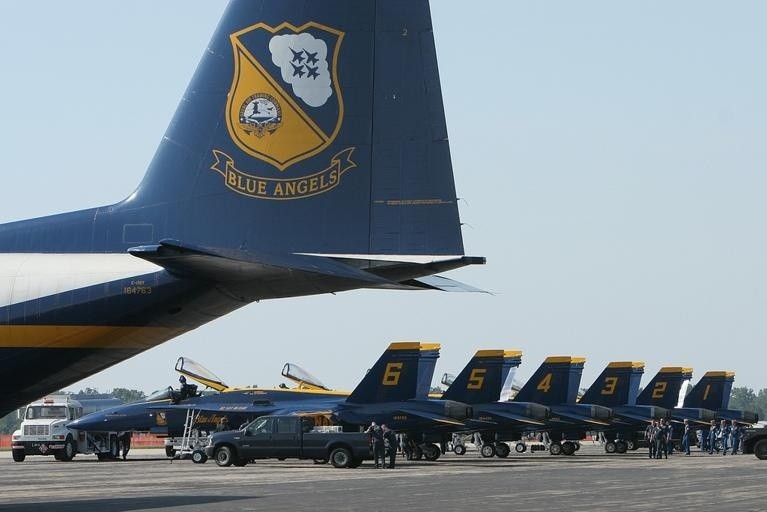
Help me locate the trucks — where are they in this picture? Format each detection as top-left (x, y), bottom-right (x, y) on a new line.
top-left (10, 393), bottom-right (135, 463)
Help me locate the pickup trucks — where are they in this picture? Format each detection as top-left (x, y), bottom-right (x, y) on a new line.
top-left (204, 414), bottom-right (390, 468)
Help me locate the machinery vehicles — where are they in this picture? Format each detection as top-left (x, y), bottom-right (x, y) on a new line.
top-left (740, 427), bottom-right (767, 460)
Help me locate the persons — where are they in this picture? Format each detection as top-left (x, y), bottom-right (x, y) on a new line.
top-left (117, 431), bottom-right (133, 460)
top-left (395, 430), bottom-right (607, 456)
top-left (644, 416), bottom-right (754, 459)
top-left (213, 417), bottom-right (229, 433)
top-left (171, 375), bottom-right (186, 400)
top-left (279, 383), bottom-right (290, 389)
top-left (110, 432), bottom-right (119, 459)
top-left (239, 416), bottom-right (257, 463)
top-left (364, 421), bottom-right (385, 469)
top-left (381, 424), bottom-right (397, 468)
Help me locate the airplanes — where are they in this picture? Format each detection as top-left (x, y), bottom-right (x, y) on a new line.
top-left (269, 339), bottom-right (758, 464)
top-left (64, 342), bottom-right (473, 464)
top-left (0, 1), bottom-right (491, 438)
top-left (174, 342), bottom-right (552, 455)
